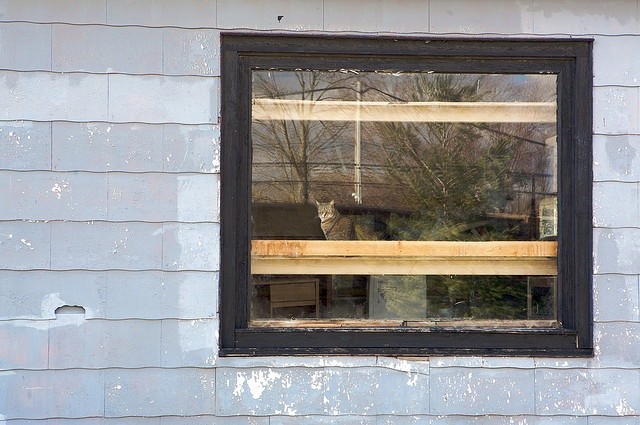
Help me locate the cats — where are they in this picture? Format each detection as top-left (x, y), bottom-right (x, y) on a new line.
top-left (314, 198), bottom-right (384, 241)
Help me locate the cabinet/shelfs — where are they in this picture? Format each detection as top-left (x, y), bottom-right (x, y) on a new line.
top-left (248, 277), bottom-right (320, 319)
top-left (330, 273), bottom-right (427, 322)
